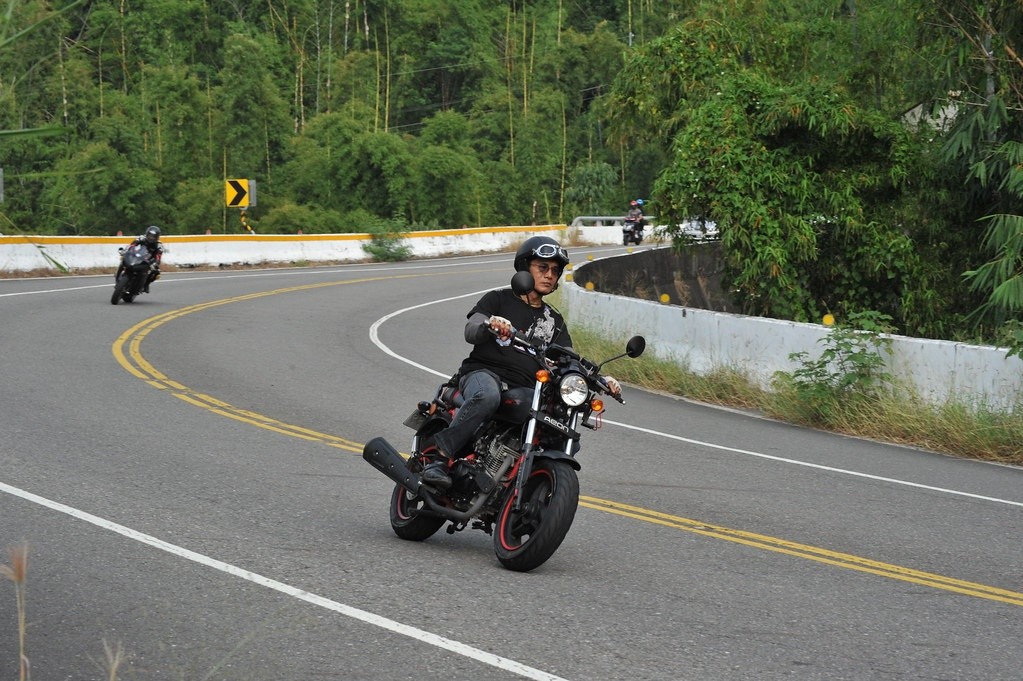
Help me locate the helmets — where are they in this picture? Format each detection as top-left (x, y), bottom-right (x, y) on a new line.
top-left (631, 200), bottom-right (638, 206)
top-left (145, 225), bottom-right (161, 245)
top-left (638, 199), bottom-right (643, 206)
top-left (513, 236), bottom-right (569, 273)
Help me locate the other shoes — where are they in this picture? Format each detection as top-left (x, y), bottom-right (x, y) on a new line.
top-left (144, 285), bottom-right (150, 294)
top-left (420, 459), bottom-right (452, 485)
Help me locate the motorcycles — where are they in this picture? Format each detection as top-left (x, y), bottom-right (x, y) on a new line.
top-left (111, 239), bottom-right (162, 306)
top-left (621, 214), bottom-right (645, 246)
top-left (361, 269), bottom-right (646, 574)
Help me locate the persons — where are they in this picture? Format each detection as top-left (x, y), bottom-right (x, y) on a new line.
top-left (422, 236), bottom-right (623, 487)
top-left (629, 199), bottom-right (644, 238)
top-left (114, 226), bottom-right (163, 293)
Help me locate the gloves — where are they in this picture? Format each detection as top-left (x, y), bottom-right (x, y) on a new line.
top-left (152, 263), bottom-right (159, 270)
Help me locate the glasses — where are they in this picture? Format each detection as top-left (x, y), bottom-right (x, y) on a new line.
top-left (535, 244), bottom-right (569, 260)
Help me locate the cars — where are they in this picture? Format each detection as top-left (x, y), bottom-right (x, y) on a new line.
top-left (674, 216), bottom-right (722, 242)
